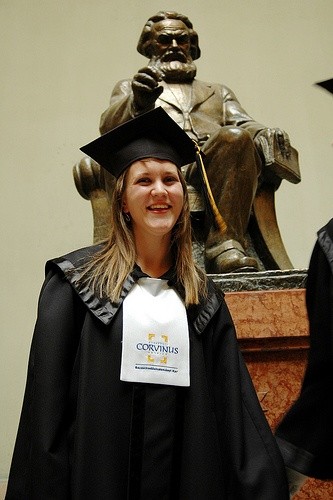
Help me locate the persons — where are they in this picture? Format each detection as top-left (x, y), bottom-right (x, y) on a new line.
top-left (99, 11), bottom-right (291, 273)
top-left (274, 217), bottom-right (333, 478)
top-left (5, 143), bottom-right (291, 500)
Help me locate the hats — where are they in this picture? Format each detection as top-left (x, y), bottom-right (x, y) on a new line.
top-left (79, 106), bottom-right (227, 233)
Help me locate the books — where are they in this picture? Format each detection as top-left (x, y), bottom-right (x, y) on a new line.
top-left (257, 130), bottom-right (301, 183)
